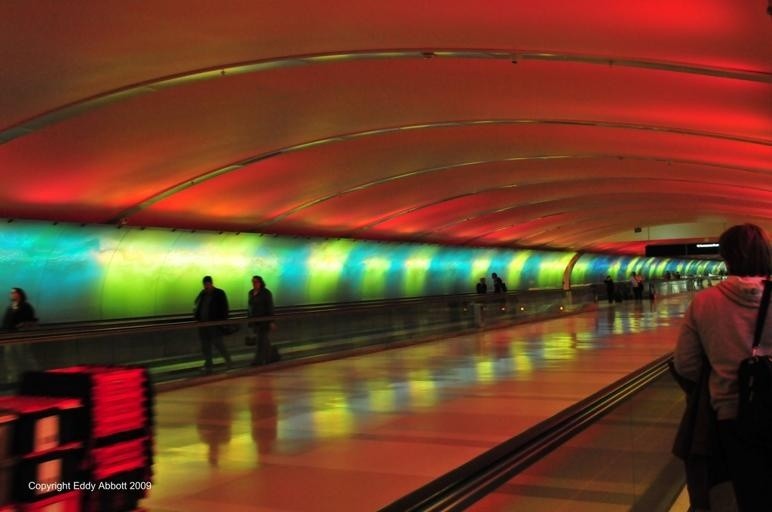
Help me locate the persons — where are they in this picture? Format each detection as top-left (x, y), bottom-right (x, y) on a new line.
top-left (577, 268), bottom-right (685, 305)
top-left (247, 279), bottom-right (277, 355)
top-left (190, 273), bottom-right (232, 364)
top-left (476, 273), bottom-right (505, 294)
top-left (5, 285), bottom-right (38, 331)
top-left (674, 223), bottom-right (772, 512)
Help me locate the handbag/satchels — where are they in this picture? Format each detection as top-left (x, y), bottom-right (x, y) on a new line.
top-left (734, 353), bottom-right (772, 455)
top-left (244, 335), bottom-right (257, 346)
